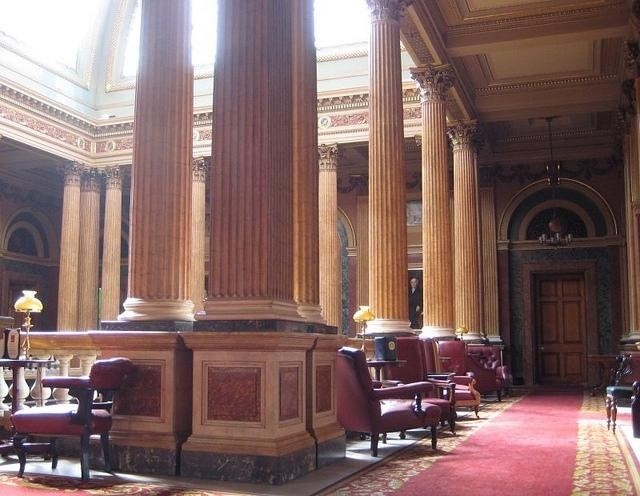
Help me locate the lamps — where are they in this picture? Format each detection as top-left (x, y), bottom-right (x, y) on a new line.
top-left (456, 326), bottom-right (468, 341)
top-left (14, 289), bottom-right (44, 359)
top-left (354, 306), bottom-right (376, 361)
top-left (539, 117), bottom-right (574, 247)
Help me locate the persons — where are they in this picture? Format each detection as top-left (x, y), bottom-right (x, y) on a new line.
top-left (409, 277), bottom-right (423, 327)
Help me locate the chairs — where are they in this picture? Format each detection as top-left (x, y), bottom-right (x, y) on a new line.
top-left (606, 352), bottom-right (640, 438)
top-left (334, 337), bottom-right (511, 457)
top-left (11, 358), bottom-right (131, 482)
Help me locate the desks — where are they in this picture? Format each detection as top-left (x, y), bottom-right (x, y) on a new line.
top-left (0, 359), bottom-right (53, 460)
